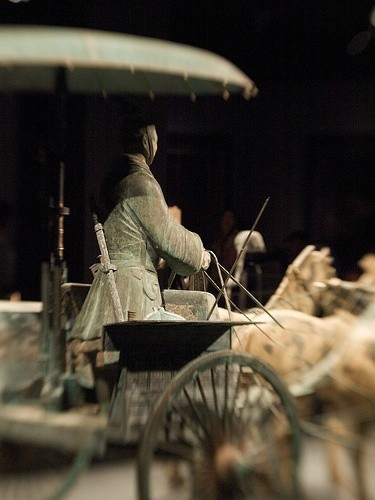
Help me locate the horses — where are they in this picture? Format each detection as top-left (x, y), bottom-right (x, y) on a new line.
top-left (219, 243), bottom-right (374, 500)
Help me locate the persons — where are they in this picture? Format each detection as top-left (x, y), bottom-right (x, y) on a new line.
top-left (72, 109), bottom-right (212, 419)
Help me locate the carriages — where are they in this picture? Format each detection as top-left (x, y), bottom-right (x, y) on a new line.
top-left (0, 18), bottom-right (375, 500)
